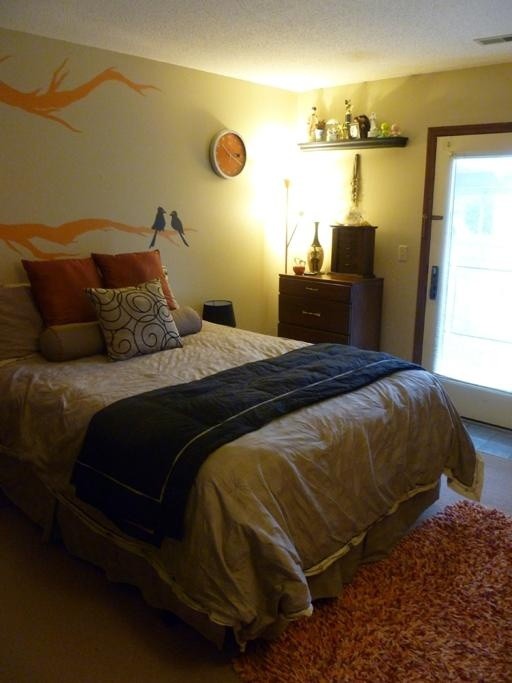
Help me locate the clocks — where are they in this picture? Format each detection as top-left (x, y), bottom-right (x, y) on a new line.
top-left (209, 127), bottom-right (249, 181)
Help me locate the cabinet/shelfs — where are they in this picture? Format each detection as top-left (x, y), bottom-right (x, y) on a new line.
top-left (276, 275), bottom-right (384, 351)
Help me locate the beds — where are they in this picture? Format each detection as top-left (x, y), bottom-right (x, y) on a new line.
top-left (0, 265), bottom-right (452, 646)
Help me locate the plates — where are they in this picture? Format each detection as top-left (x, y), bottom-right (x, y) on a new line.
top-left (304, 272), bottom-right (318, 275)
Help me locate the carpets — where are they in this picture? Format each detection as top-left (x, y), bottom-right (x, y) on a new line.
top-left (226, 497), bottom-right (512, 682)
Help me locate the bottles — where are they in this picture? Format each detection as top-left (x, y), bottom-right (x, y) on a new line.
top-left (309, 97), bottom-right (401, 142)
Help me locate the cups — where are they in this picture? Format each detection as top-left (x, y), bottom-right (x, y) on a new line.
top-left (293, 266), bottom-right (305, 275)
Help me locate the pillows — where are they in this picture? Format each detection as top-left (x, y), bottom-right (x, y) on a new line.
top-left (21, 249), bottom-right (203, 361)
